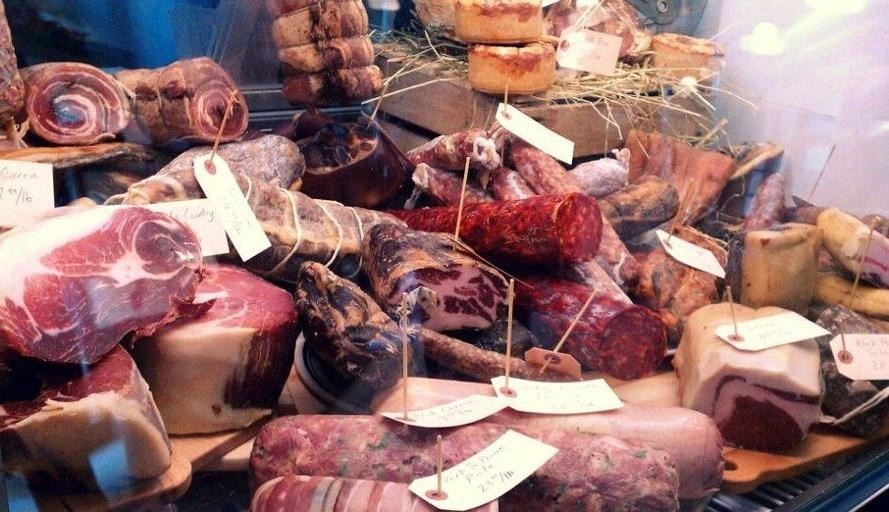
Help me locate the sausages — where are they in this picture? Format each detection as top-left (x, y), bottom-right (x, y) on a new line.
top-left (381, 127), bottom-right (680, 380)
top-left (740, 171), bottom-right (786, 236)
